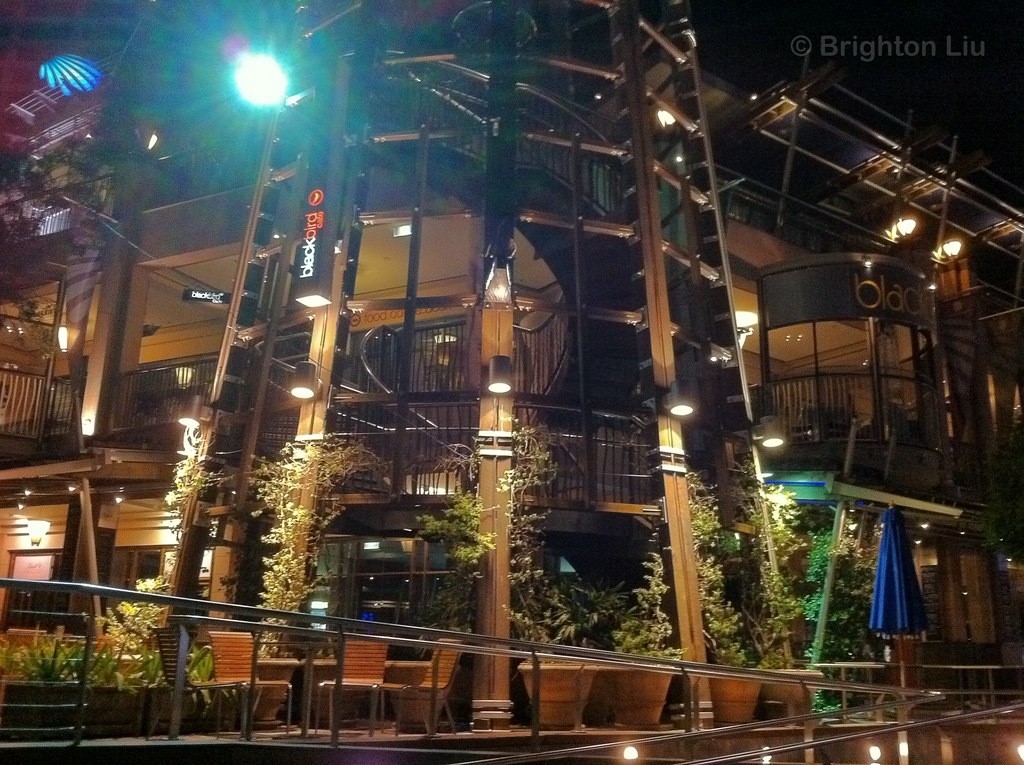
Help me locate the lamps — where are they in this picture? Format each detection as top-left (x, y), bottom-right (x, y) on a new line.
top-left (58, 327), bottom-right (69, 352)
top-left (291, 239), bottom-right (333, 310)
top-left (485, 355), bottom-right (515, 395)
top-left (662, 379), bottom-right (697, 417)
top-left (289, 361), bottom-right (321, 401)
top-left (27, 519), bottom-right (52, 547)
top-left (175, 366), bottom-right (196, 390)
top-left (753, 412), bottom-right (788, 453)
top-left (861, 257), bottom-right (872, 268)
top-left (178, 394), bottom-right (212, 428)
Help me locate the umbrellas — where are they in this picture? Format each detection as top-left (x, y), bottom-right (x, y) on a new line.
top-left (869, 507), bottom-right (929, 689)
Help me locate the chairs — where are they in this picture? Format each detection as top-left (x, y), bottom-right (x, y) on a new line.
top-left (382, 640), bottom-right (466, 735)
top-left (317, 640), bottom-right (390, 737)
top-left (206, 631), bottom-right (291, 740)
top-left (151, 625), bottom-right (249, 739)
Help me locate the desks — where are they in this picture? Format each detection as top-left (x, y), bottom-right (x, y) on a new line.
top-left (246, 638), bottom-right (357, 739)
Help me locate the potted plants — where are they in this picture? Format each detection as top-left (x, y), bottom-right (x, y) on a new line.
top-left (140, 644), bottom-right (192, 733)
top-left (78, 576), bottom-right (146, 733)
top-left (697, 558), bottom-right (759, 727)
top-left (4, 638), bottom-right (83, 734)
top-left (511, 599), bottom-right (594, 732)
top-left (747, 522), bottom-right (825, 720)
top-left (600, 552), bottom-right (686, 727)
